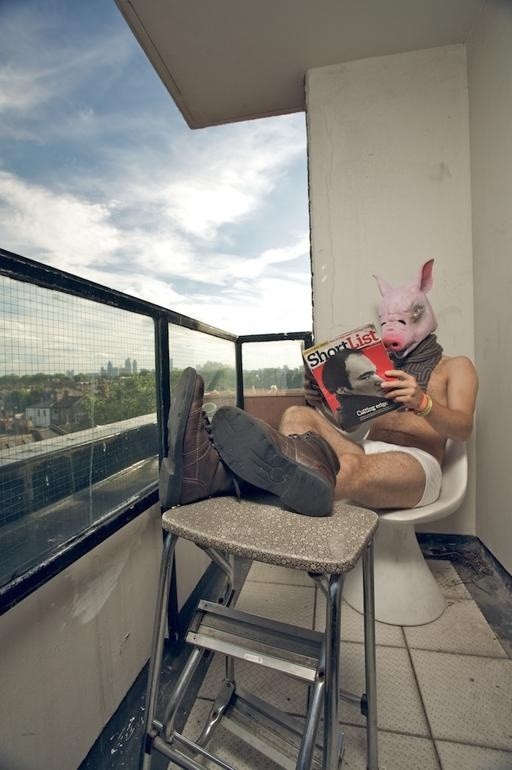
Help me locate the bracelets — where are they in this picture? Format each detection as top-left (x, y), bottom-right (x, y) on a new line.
top-left (415, 394), bottom-right (428, 413)
top-left (416, 395), bottom-right (433, 416)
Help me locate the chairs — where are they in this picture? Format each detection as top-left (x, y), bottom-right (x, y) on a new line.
top-left (339, 417), bottom-right (471, 628)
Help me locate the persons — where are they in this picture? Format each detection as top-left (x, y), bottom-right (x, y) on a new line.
top-left (322, 349), bottom-right (402, 430)
top-left (158, 259), bottom-right (479, 517)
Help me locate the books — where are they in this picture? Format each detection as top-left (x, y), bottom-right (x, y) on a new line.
top-left (302, 321), bottom-right (406, 431)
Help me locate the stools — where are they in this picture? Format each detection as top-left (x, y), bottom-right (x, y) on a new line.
top-left (140, 496), bottom-right (380, 770)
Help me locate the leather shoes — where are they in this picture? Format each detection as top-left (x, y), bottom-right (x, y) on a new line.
top-left (159, 367), bottom-right (246, 506)
top-left (212, 406), bottom-right (339, 516)
